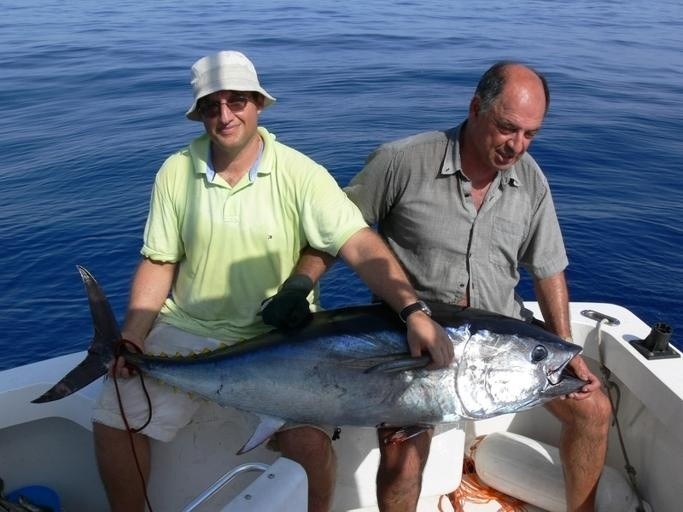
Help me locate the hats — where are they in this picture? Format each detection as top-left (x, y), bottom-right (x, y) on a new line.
top-left (185, 51), bottom-right (276, 121)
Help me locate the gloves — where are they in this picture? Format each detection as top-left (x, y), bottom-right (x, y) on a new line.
top-left (256, 274), bottom-right (313, 328)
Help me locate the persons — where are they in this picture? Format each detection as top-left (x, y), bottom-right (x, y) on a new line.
top-left (91, 51), bottom-right (455, 512)
top-left (258, 61), bottom-right (613, 512)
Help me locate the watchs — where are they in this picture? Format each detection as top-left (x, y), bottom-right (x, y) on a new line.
top-left (397, 300), bottom-right (433, 329)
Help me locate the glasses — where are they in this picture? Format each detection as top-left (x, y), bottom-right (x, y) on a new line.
top-left (201, 96), bottom-right (253, 114)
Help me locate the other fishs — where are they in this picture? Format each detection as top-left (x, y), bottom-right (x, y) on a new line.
top-left (29, 263), bottom-right (592, 455)
